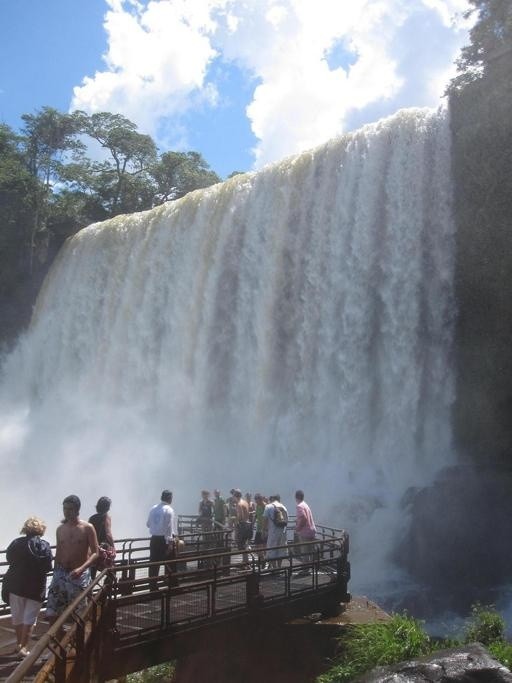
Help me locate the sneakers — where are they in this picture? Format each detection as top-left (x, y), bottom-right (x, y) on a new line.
top-left (15, 644), bottom-right (30, 660)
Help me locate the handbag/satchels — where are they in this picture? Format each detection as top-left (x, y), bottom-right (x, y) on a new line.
top-left (2, 568), bottom-right (12, 604)
top-left (95, 543), bottom-right (116, 570)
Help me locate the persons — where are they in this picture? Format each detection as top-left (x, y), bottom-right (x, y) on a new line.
top-left (261, 493), bottom-right (289, 570)
top-left (41, 494), bottom-right (101, 663)
top-left (198, 486), bottom-right (271, 564)
top-left (86, 495), bottom-right (116, 587)
top-left (4, 516), bottom-right (55, 661)
top-left (292, 489), bottom-right (318, 574)
top-left (145, 488), bottom-right (180, 591)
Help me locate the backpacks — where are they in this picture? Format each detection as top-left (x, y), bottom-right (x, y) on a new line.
top-left (274, 507), bottom-right (287, 527)
